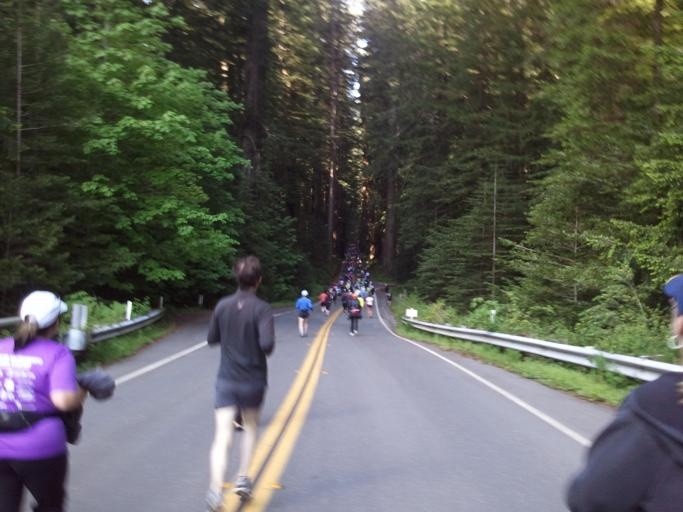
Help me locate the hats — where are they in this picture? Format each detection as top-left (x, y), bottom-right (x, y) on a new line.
top-left (20, 290), bottom-right (68, 329)
top-left (301, 290), bottom-right (308, 296)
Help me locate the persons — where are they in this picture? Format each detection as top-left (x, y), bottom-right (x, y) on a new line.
top-left (205, 255), bottom-right (274, 511)
top-left (294, 289), bottom-right (314, 337)
top-left (235, 411), bottom-right (242, 431)
top-left (563, 269), bottom-right (683, 511)
top-left (0, 289), bottom-right (87, 512)
top-left (319, 245), bottom-right (375, 336)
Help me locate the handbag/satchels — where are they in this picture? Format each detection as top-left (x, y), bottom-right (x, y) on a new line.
top-left (1, 411), bottom-right (39, 431)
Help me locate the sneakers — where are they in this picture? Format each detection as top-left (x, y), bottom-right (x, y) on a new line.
top-left (233, 476), bottom-right (253, 499)
